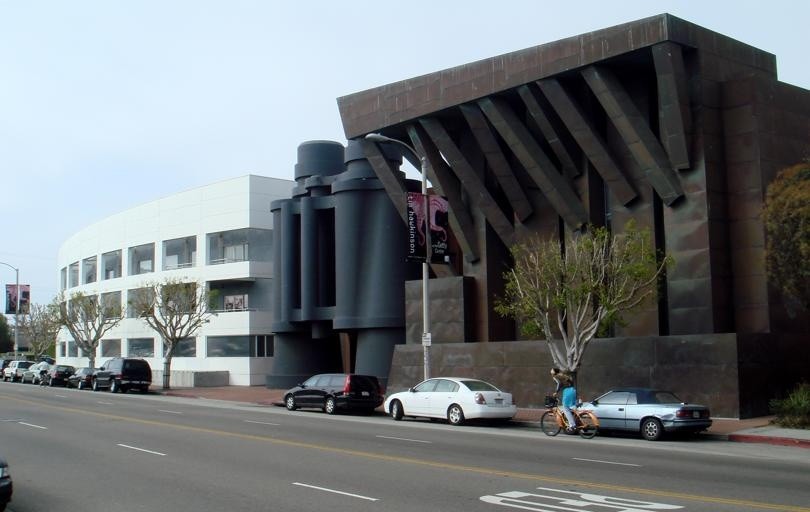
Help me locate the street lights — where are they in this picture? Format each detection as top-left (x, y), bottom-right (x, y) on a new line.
top-left (365, 132), bottom-right (432, 381)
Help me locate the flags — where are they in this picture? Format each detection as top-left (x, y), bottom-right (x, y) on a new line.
top-left (404, 190), bottom-right (428, 262)
top-left (4, 283), bottom-right (18, 314)
top-left (18, 283), bottom-right (31, 315)
top-left (428, 194), bottom-right (451, 265)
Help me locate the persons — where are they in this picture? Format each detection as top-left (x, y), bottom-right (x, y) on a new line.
top-left (36, 358), bottom-right (50, 381)
top-left (551, 367), bottom-right (578, 432)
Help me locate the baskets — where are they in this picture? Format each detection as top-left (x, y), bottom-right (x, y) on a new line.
top-left (544, 395), bottom-right (558, 408)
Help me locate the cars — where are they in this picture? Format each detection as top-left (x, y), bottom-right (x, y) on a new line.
top-left (0, 455), bottom-right (13, 512)
top-left (0, 356), bottom-right (152, 394)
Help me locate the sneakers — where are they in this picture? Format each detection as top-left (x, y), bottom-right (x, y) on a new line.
top-left (566, 426), bottom-right (576, 432)
